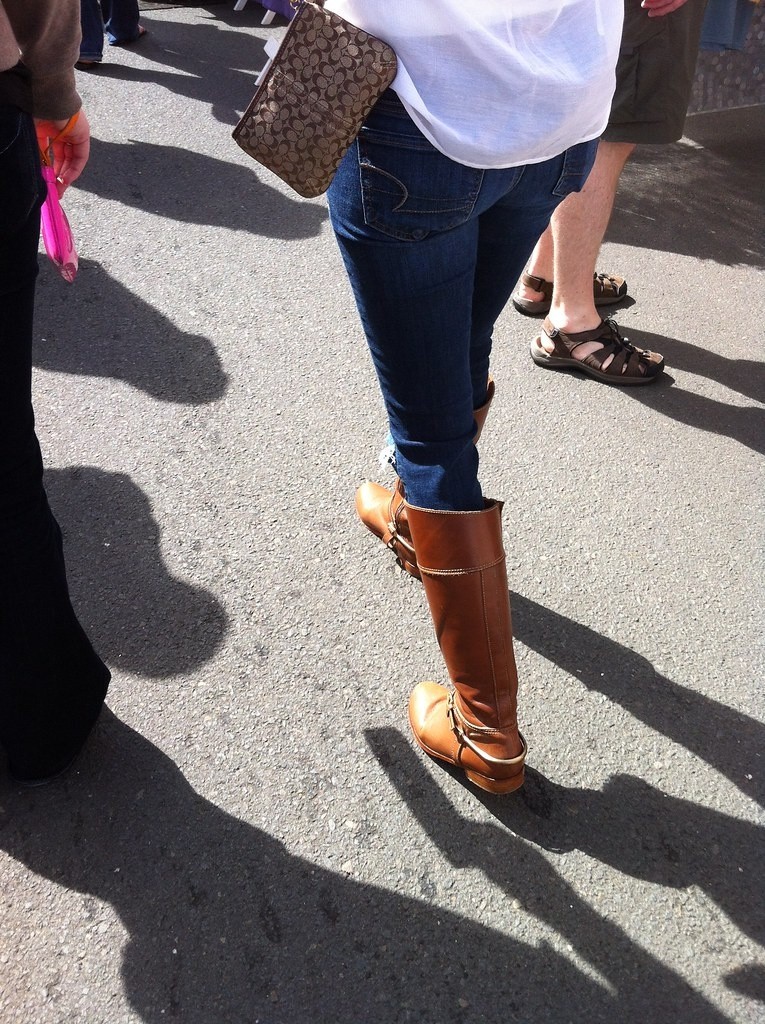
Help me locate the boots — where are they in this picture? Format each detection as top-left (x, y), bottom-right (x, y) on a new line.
top-left (353, 381), bottom-right (495, 582)
top-left (400, 497), bottom-right (529, 795)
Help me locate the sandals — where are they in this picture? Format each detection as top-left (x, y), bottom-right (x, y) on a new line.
top-left (529, 314), bottom-right (665, 387)
top-left (512, 269), bottom-right (627, 316)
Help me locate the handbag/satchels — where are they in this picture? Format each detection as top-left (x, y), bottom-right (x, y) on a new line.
top-left (230, 0), bottom-right (397, 199)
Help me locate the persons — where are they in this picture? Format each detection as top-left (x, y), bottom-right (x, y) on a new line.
top-left (1, 1), bottom-right (159, 789)
top-left (316, 0), bottom-right (627, 797)
top-left (508, 1), bottom-right (706, 386)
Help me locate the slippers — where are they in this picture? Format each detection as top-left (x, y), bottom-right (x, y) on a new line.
top-left (135, 23), bottom-right (148, 39)
top-left (72, 59), bottom-right (98, 70)
